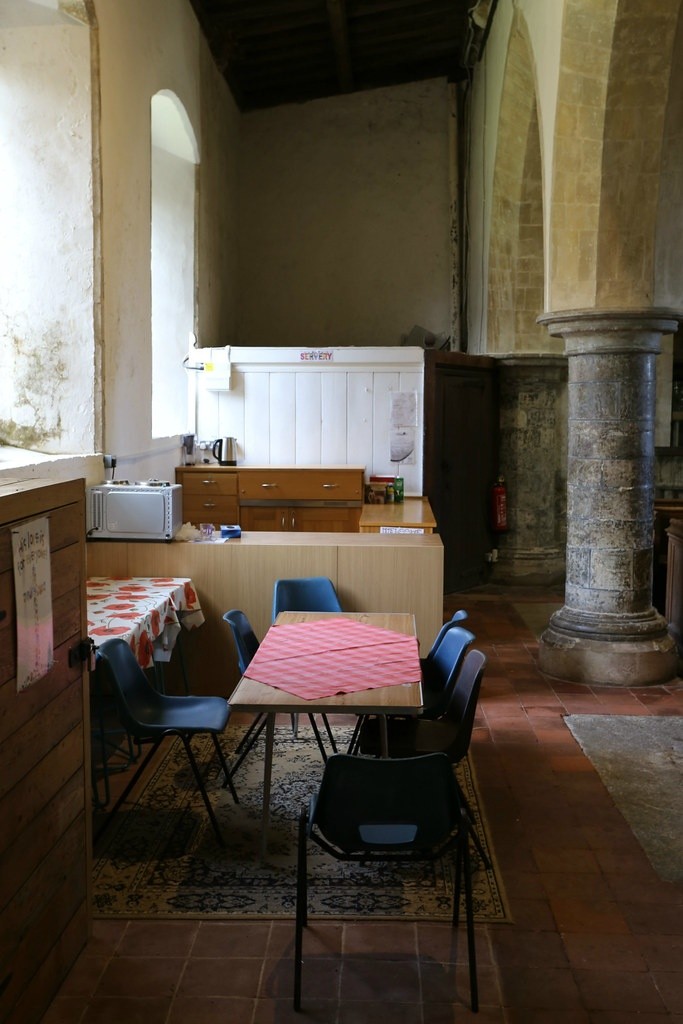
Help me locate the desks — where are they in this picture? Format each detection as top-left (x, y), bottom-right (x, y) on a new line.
top-left (86, 575), bottom-right (205, 801)
top-left (227, 610), bottom-right (424, 853)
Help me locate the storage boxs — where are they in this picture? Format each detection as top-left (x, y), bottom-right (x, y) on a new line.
top-left (365, 482), bottom-right (387, 504)
top-left (370, 477), bottom-right (395, 502)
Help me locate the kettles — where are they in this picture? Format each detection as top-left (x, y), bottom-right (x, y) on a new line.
top-left (212, 436), bottom-right (237, 467)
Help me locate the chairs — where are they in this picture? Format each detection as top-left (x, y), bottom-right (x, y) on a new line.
top-left (96, 579), bottom-right (493, 1013)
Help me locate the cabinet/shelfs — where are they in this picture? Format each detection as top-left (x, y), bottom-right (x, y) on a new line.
top-left (87, 347), bottom-right (444, 658)
top-left (0, 477), bottom-right (98, 1024)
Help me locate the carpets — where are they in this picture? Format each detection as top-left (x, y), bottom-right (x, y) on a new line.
top-left (95, 724), bottom-right (519, 924)
